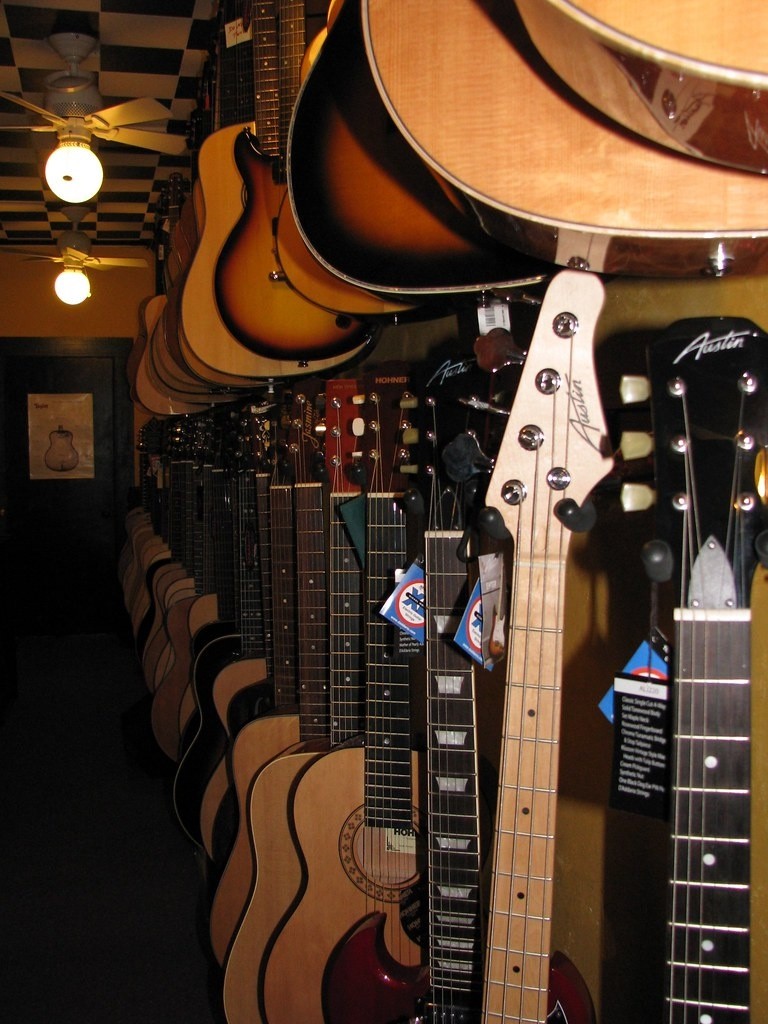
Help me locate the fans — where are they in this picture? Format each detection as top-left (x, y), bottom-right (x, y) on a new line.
top-left (7, 205), bottom-right (150, 270)
top-left (1, 33), bottom-right (187, 157)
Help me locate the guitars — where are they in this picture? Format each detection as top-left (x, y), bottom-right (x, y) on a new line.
top-left (615, 313), bottom-right (767, 1024)
top-left (213, 0), bottom-right (373, 354)
top-left (321, 335), bottom-right (600, 1024)
top-left (257, 356), bottom-right (425, 1022)
top-left (287, 2), bottom-right (553, 303)
top-left (114, 0), bottom-right (370, 1022)
top-left (347, 1), bottom-right (768, 279)
top-left (441, 255), bottom-right (618, 1023)
top-left (274, 1), bottom-right (456, 323)
top-left (512, 0), bottom-right (768, 169)
